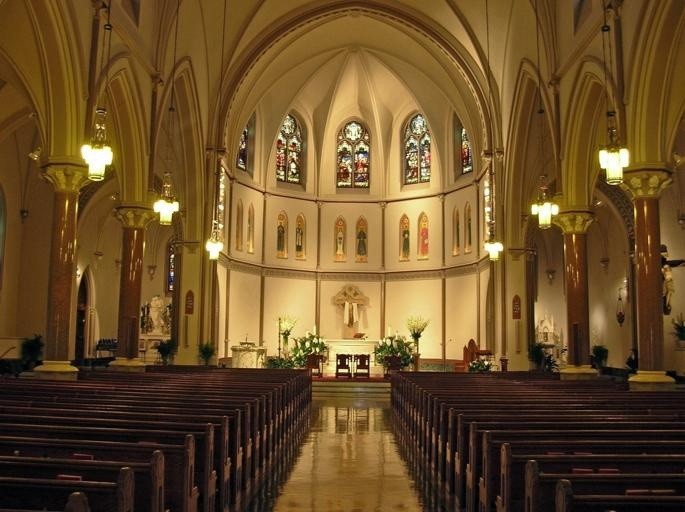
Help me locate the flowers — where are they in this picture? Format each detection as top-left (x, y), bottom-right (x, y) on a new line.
top-left (273, 312), bottom-right (298, 351)
top-left (404, 314), bottom-right (430, 353)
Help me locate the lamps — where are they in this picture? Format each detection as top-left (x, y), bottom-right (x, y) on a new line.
top-left (599, 4), bottom-right (630, 186)
top-left (483, 169), bottom-right (504, 261)
top-left (81, 25), bottom-right (114, 182)
top-left (206, 165), bottom-right (223, 260)
top-left (615, 285), bottom-right (626, 327)
top-left (531, 104), bottom-right (560, 230)
top-left (153, 104), bottom-right (179, 226)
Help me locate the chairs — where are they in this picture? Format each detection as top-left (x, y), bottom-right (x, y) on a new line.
top-left (335, 353), bottom-right (353, 380)
top-left (383, 355), bottom-right (401, 378)
top-left (306, 354), bottom-right (324, 379)
top-left (353, 354), bottom-right (371, 380)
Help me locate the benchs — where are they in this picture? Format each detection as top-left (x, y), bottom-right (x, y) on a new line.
top-left (388, 369), bottom-right (685, 512)
top-left (1, 361), bottom-right (312, 512)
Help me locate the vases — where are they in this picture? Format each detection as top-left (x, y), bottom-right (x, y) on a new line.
top-left (282, 351), bottom-right (290, 360)
top-left (412, 353), bottom-right (421, 370)
